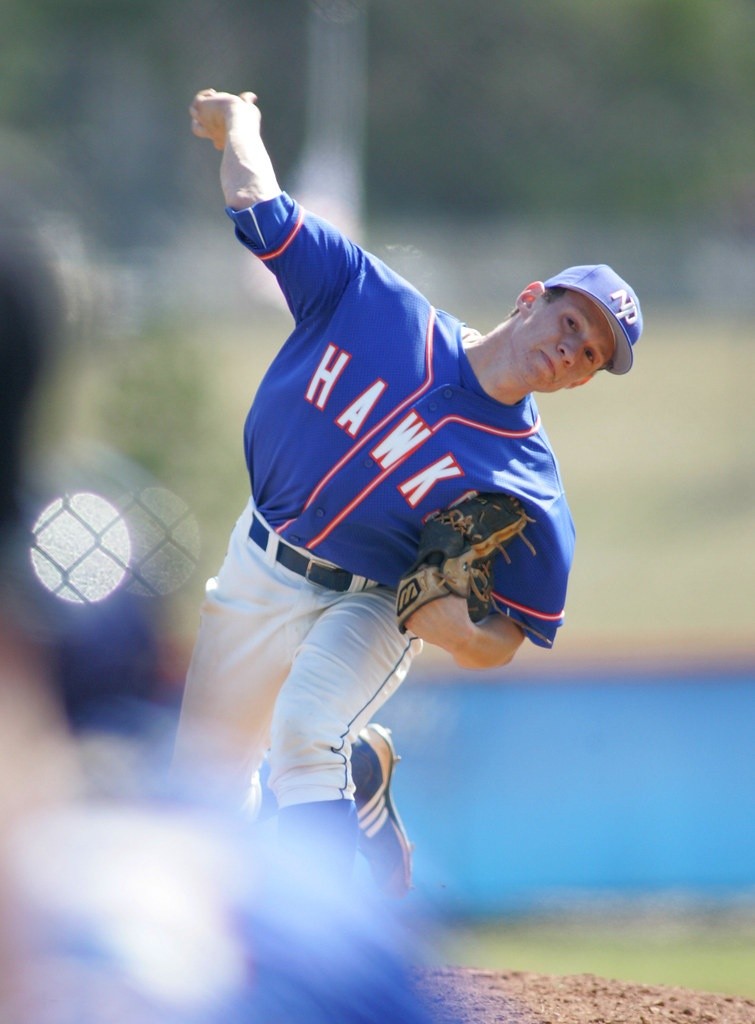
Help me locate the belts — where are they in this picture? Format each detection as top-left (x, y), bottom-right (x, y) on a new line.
top-left (248, 513), bottom-right (386, 591)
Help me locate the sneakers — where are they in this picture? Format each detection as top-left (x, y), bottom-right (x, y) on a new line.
top-left (350, 724), bottom-right (413, 896)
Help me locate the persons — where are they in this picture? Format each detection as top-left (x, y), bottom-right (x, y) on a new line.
top-left (187, 89), bottom-right (643, 900)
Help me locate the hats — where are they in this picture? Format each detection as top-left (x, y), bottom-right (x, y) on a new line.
top-left (543, 264), bottom-right (643, 376)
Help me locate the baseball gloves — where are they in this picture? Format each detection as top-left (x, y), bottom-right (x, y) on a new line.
top-left (393, 491), bottom-right (530, 636)
